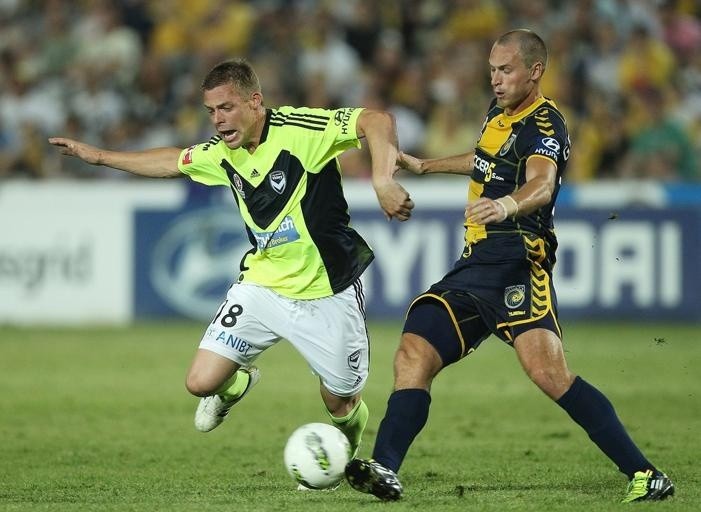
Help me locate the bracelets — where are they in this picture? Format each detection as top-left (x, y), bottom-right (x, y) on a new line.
top-left (494, 196), bottom-right (518, 223)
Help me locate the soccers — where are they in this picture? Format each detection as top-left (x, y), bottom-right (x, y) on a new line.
top-left (283, 423), bottom-right (354, 492)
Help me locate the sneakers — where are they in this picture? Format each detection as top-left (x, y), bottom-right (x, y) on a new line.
top-left (345, 458), bottom-right (403, 501)
top-left (622, 469), bottom-right (674, 503)
top-left (195, 366), bottom-right (260, 432)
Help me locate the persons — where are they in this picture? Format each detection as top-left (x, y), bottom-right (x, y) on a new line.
top-left (346, 29), bottom-right (675, 506)
top-left (49, 60), bottom-right (415, 493)
